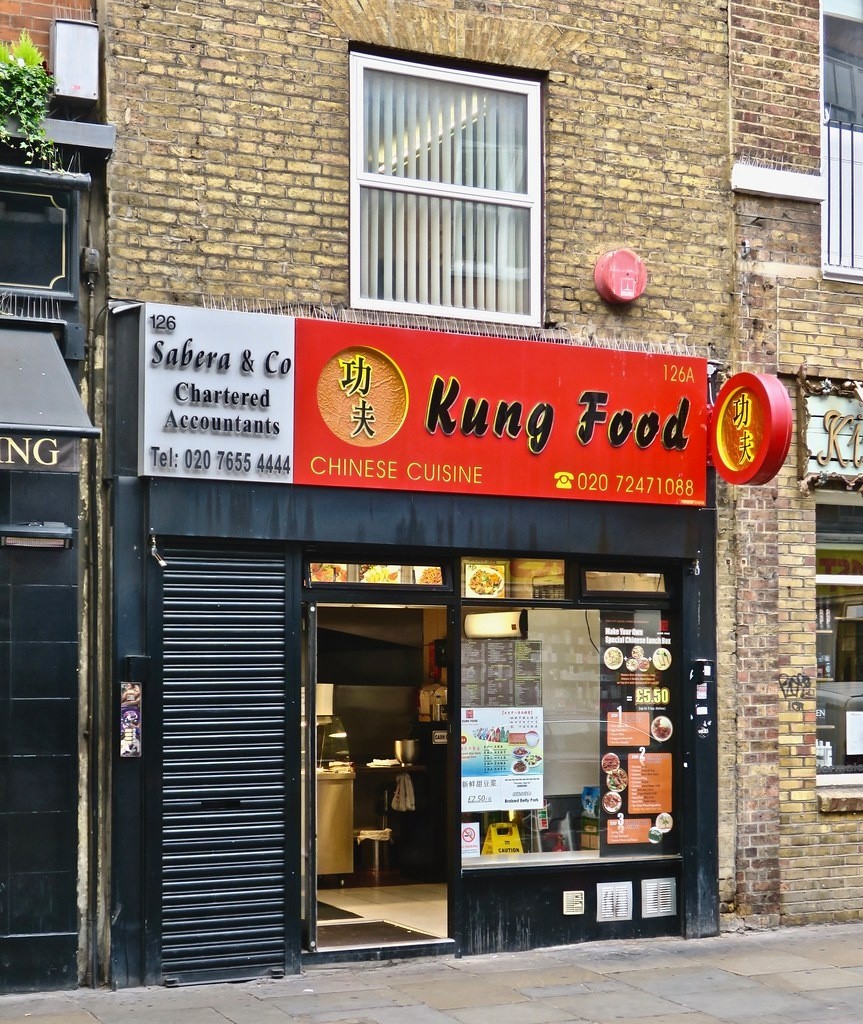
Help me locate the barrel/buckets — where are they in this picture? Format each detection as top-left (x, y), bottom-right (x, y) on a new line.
top-left (360, 830), bottom-right (392, 879)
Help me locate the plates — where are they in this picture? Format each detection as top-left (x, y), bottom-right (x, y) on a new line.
top-left (521, 755), bottom-right (542, 767)
top-left (626, 646), bottom-right (649, 671)
top-left (604, 793), bottom-right (622, 813)
top-left (652, 648), bottom-right (672, 671)
top-left (607, 768), bottom-right (628, 792)
top-left (651, 716), bottom-right (673, 741)
top-left (510, 760), bottom-right (529, 774)
top-left (604, 647), bottom-right (623, 670)
top-left (601, 753), bottom-right (620, 774)
top-left (467, 568), bottom-right (503, 598)
top-left (512, 747), bottom-right (529, 757)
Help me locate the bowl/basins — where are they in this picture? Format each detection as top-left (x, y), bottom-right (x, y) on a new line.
top-left (395, 739), bottom-right (418, 767)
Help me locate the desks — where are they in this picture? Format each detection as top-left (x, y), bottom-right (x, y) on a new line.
top-left (356, 764), bottom-right (427, 877)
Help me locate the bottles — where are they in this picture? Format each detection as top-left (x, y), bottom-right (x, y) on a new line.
top-left (500, 727), bottom-right (505, 742)
top-left (496, 728), bottom-right (500, 742)
top-left (817, 651), bottom-right (831, 678)
top-left (479, 727), bottom-right (495, 742)
top-left (506, 730), bottom-right (509, 742)
top-left (817, 596), bottom-right (831, 630)
top-left (433, 695), bottom-right (441, 722)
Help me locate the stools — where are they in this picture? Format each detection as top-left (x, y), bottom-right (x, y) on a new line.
top-left (353, 827), bottom-right (393, 879)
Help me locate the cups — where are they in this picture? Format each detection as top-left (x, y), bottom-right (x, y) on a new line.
top-left (526, 734), bottom-right (539, 747)
